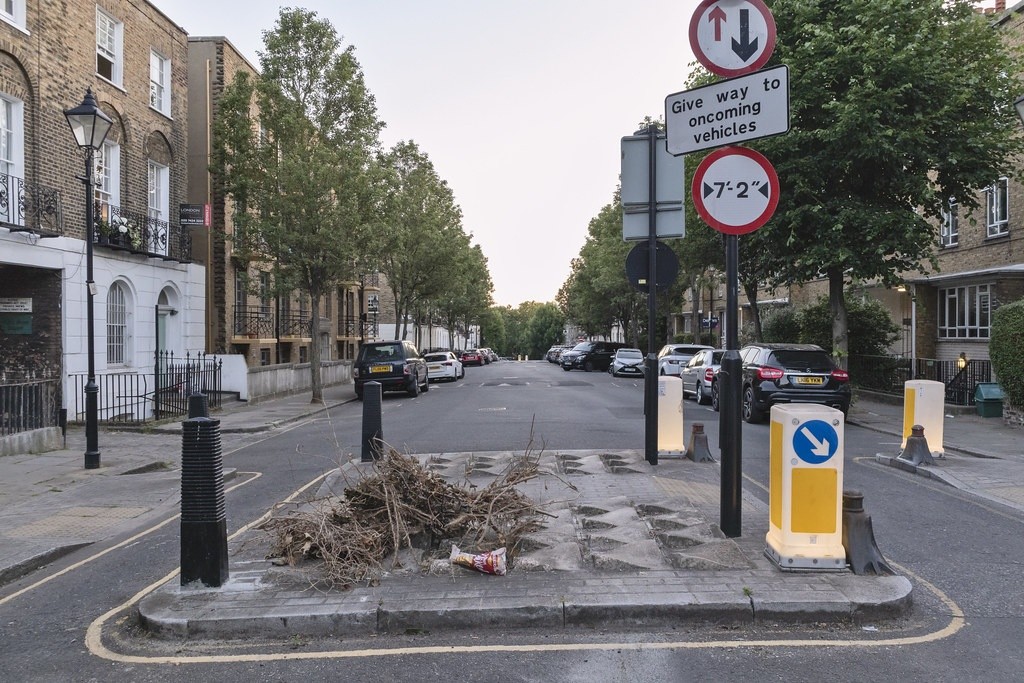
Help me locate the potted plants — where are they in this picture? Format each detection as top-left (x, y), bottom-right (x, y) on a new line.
top-left (98, 220), bottom-right (120, 244)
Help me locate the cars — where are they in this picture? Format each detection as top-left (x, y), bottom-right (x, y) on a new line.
top-left (546, 341), bottom-right (575, 368)
top-left (423, 352), bottom-right (465, 382)
top-left (610, 348), bottom-right (647, 378)
top-left (710, 343), bottom-right (851, 423)
top-left (679, 348), bottom-right (726, 404)
top-left (460, 348), bottom-right (499, 366)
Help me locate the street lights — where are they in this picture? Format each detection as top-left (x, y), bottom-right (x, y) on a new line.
top-left (371, 296), bottom-right (379, 340)
top-left (61, 86), bottom-right (114, 469)
top-left (708, 264), bottom-right (716, 346)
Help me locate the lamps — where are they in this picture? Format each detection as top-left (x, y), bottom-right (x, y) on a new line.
top-left (958, 352), bottom-right (966, 367)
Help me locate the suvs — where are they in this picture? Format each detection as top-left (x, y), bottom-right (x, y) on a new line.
top-left (353, 339), bottom-right (429, 400)
top-left (658, 344), bottom-right (716, 378)
top-left (557, 340), bottom-right (634, 374)
